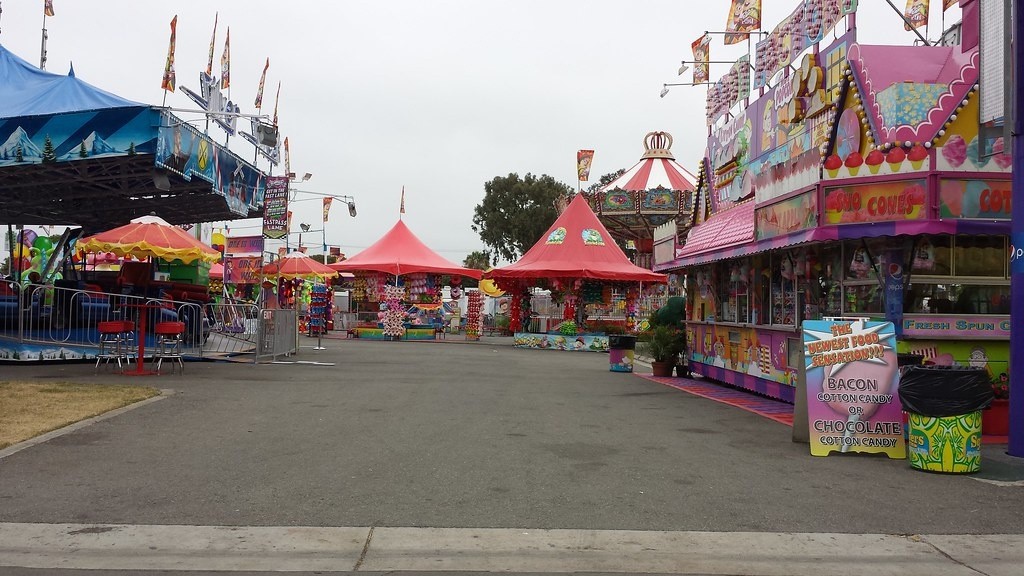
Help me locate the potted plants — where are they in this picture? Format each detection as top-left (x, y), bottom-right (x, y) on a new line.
top-left (647, 297), bottom-right (688, 377)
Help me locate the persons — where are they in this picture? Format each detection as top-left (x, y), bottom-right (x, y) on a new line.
top-left (206, 295), bottom-right (216, 326)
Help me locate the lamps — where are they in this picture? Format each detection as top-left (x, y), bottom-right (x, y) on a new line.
top-left (679, 61), bottom-right (737, 75)
top-left (292, 173), bottom-right (312, 183)
top-left (291, 189), bottom-right (356, 217)
top-left (700, 31), bottom-right (769, 48)
top-left (660, 82), bottom-right (716, 97)
top-left (299, 219), bottom-right (312, 231)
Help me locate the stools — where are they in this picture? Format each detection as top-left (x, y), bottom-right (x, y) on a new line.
top-left (95, 321), bottom-right (137, 371)
top-left (151, 323), bottom-right (185, 376)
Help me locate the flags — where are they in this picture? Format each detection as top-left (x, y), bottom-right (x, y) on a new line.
top-left (220, 26), bottom-right (230, 90)
top-left (206, 12), bottom-right (219, 76)
top-left (273, 81), bottom-right (280, 125)
top-left (691, 32), bottom-right (709, 87)
top-left (284, 137), bottom-right (290, 178)
top-left (288, 211), bottom-right (292, 235)
top-left (724, 0), bottom-right (762, 45)
top-left (399, 185), bottom-right (405, 214)
top-left (161, 15), bottom-right (178, 93)
top-left (254, 57), bottom-right (270, 108)
top-left (578, 150), bottom-right (595, 182)
top-left (904, 0), bottom-right (930, 31)
top-left (41, 0), bottom-right (56, 18)
top-left (323, 197), bottom-right (333, 222)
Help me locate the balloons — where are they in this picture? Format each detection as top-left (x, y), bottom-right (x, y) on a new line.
top-left (14, 229), bottom-right (52, 272)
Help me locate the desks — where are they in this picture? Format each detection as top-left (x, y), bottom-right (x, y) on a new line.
top-left (114, 304), bottom-right (176, 376)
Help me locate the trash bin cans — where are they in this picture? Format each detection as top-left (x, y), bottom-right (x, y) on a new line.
top-left (608, 334), bottom-right (637, 372)
top-left (897, 364), bottom-right (996, 474)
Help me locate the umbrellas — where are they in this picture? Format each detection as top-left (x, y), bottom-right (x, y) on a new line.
top-left (76, 210), bottom-right (222, 264)
top-left (255, 251), bottom-right (337, 304)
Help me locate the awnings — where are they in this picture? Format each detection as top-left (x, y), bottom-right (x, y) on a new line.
top-left (678, 197), bottom-right (757, 258)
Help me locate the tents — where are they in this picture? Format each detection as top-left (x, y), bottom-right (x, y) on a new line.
top-left (485, 193), bottom-right (668, 296)
top-left (324, 220), bottom-right (482, 287)
top-left (0, 43), bottom-right (267, 227)
